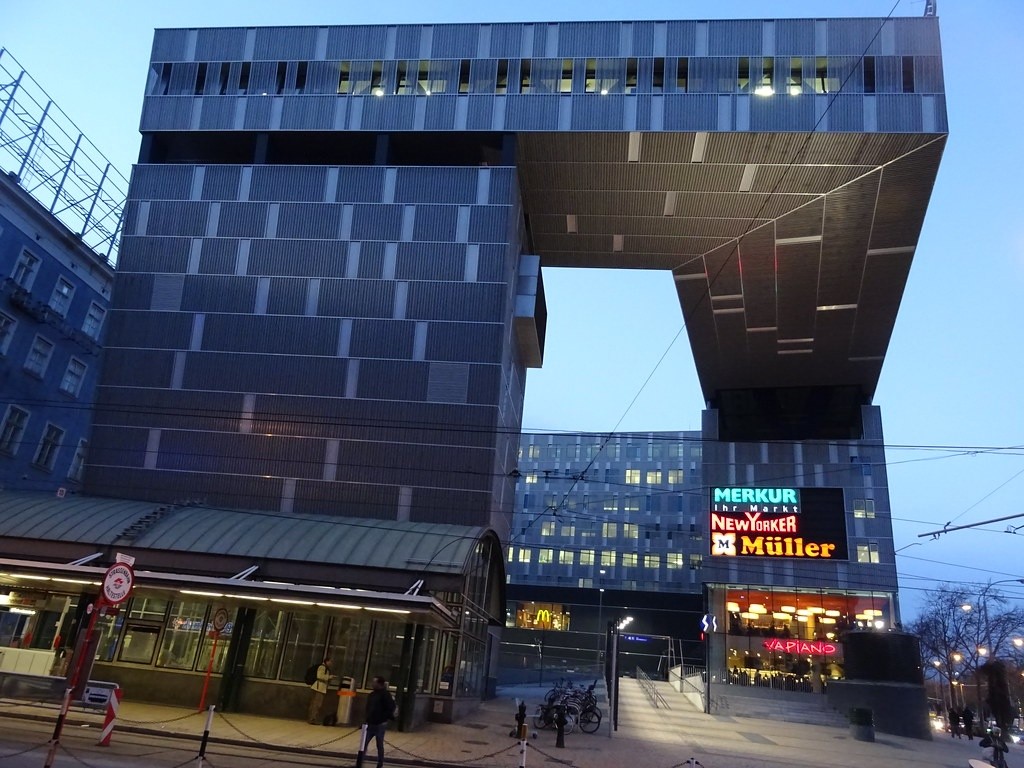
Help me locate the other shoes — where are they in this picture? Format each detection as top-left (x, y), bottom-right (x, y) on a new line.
top-left (308, 719), bottom-right (322, 725)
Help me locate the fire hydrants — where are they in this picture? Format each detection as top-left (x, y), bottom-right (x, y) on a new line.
top-left (510, 700), bottom-right (527, 739)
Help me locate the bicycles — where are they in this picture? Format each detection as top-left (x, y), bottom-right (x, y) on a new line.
top-left (533, 676), bottom-right (603, 735)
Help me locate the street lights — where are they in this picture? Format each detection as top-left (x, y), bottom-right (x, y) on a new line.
top-left (934, 605), bottom-right (971, 732)
top-left (934, 653), bottom-right (961, 707)
top-left (975, 647), bottom-right (986, 736)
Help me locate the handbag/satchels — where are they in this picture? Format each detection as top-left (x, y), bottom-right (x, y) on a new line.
top-left (981, 735), bottom-right (994, 761)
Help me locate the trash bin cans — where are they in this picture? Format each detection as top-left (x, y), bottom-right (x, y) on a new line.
top-left (335, 676), bottom-right (355, 726)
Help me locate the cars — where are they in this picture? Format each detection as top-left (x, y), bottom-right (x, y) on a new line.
top-left (929, 715), bottom-right (1024, 747)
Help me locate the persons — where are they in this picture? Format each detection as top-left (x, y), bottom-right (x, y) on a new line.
top-left (962, 706), bottom-right (974, 740)
top-left (308, 658), bottom-right (335, 725)
top-left (979, 727), bottom-right (1009, 768)
top-left (948, 708), bottom-right (961, 739)
top-left (356, 676), bottom-right (397, 768)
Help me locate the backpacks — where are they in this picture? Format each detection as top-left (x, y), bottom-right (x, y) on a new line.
top-left (304, 664), bottom-right (327, 686)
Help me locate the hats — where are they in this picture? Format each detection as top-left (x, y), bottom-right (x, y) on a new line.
top-left (991, 726), bottom-right (1002, 734)
